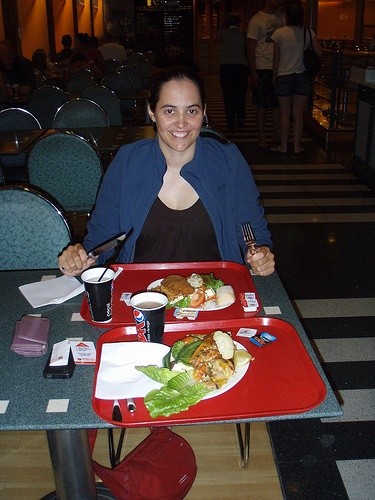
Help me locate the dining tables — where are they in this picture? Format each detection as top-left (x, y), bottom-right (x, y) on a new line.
top-left (0, 270), bottom-right (346, 500)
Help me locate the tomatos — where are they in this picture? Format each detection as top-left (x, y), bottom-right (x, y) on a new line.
top-left (181, 335), bottom-right (195, 344)
top-left (189, 290), bottom-right (206, 308)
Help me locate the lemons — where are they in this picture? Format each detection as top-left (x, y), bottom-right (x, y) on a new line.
top-left (233, 349), bottom-right (252, 366)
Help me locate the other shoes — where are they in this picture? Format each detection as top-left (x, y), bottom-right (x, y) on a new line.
top-left (259, 140), bottom-right (268, 146)
top-left (236, 120), bottom-right (246, 128)
top-left (272, 137), bottom-right (281, 143)
top-left (228, 122), bottom-right (235, 129)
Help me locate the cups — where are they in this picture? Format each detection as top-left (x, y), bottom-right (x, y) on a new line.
top-left (130, 292), bottom-right (168, 343)
top-left (80, 267), bottom-right (114, 323)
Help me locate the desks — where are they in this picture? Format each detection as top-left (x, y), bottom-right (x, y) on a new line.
top-left (0, 130), bottom-right (47, 185)
top-left (25, 128), bottom-right (156, 172)
top-left (118, 88), bottom-right (151, 125)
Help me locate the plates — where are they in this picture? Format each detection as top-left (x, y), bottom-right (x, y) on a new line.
top-left (147, 278), bottom-right (236, 311)
top-left (156, 340), bottom-right (249, 400)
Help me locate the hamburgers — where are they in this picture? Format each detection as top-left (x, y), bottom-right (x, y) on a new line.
top-left (150, 274), bottom-right (189, 300)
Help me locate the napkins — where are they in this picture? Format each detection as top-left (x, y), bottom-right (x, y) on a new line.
top-left (19, 274), bottom-right (85, 308)
top-left (96, 342), bottom-right (172, 400)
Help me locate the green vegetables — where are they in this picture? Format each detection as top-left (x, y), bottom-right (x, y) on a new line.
top-left (166, 272), bottom-right (224, 309)
top-left (135, 340), bottom-right (213, 419)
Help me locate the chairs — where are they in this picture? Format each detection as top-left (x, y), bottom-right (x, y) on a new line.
top-left (0, 51), bottom-right (150, 272)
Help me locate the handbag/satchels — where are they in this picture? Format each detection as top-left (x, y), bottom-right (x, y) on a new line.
top-left (87, 428), bottom-right (197, 500)
top-left (303, 26), bottom-right (321, 75)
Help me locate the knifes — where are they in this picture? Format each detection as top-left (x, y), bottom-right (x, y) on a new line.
top-left (62, 234), bottom-right (128, 270)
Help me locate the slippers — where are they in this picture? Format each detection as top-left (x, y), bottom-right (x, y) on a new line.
top-left (273, 146), bottom-right (288, 155)
top-left (294, 145), bottom-right (307, 154)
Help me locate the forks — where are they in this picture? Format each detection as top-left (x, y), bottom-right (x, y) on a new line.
top-left (240, 224), bottom-right (258, 255)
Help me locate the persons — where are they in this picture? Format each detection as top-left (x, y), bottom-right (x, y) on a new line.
top-left (57, 68), bottom-right (275, 276)
top-left (0, 0), bottom-right (323, 155)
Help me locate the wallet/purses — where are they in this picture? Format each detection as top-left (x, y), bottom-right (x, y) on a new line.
top-left (11, 315), bottom-right (51, 357)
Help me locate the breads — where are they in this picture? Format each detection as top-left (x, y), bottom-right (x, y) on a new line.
top-left (215, 285), bottom-right (235, 306)
top-left (212, 330), bottom-right (235, 360)
